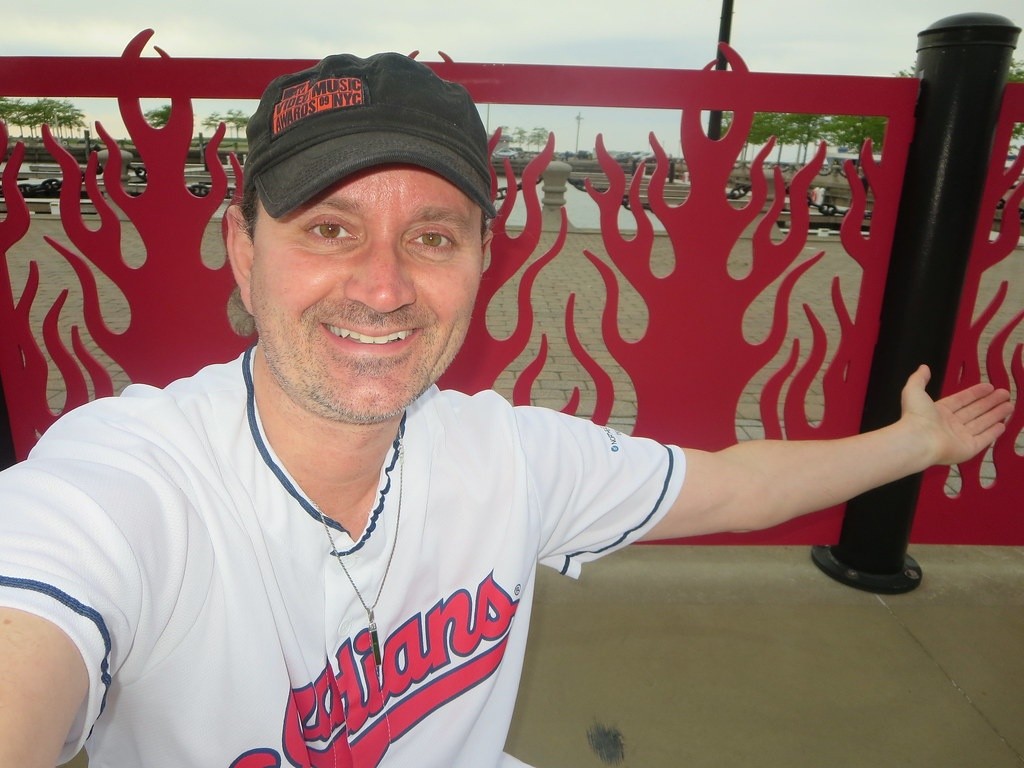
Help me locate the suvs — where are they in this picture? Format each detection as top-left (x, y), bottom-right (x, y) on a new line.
top-left (509, 147), bottom-right (524, 159)
top-left (577, 151), bottom-right (592, 160)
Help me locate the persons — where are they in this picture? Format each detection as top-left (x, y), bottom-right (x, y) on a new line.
top-left (0, 52), bottom-right (1014, 768)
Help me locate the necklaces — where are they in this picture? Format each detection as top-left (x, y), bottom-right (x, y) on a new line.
top-left (305, 420), bottom-right (406, 664)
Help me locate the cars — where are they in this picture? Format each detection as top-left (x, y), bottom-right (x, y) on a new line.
top-left (494, 148), bottom-right (519, 160)
top-left (611, 150), bottom-right (657, 164)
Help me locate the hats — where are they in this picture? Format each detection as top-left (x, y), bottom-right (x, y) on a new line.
top-left (242, 51), bottom-right (498, 220)
